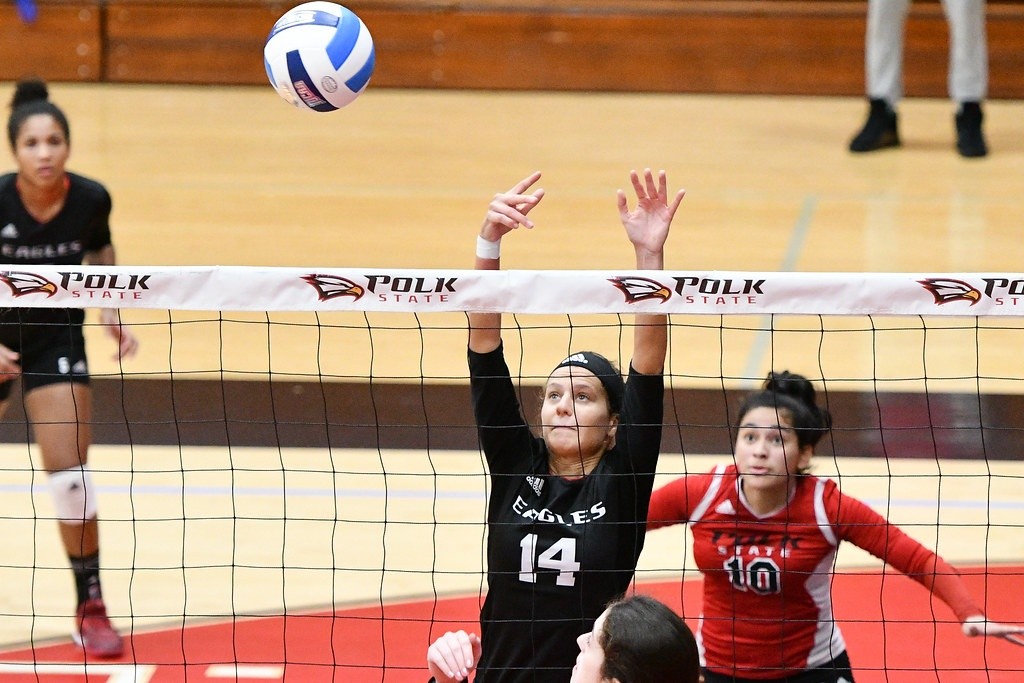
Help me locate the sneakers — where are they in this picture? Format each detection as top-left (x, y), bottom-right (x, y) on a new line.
top-left (850, 98), bottom-right (899, 151)
top-left (73, 598), bottom-right (124, 658)
top-left (956, 102), bottom-right (985, 157)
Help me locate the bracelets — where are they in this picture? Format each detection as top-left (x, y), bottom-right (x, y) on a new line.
top-left (477, 235), bottom-right (500, 259)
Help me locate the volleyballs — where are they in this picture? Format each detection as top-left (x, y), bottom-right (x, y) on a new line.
top-left (262, 0), bottom-right (378, 117)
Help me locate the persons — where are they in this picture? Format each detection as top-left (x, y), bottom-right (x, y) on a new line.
top-left (0, 78), bottom-right (137, 657)
top-left (467, 168), bottom-right (685, 683)
top-left (850, 0), bottom-right (988, 157)
top-left (427, 595), bottom-right (700, 683)
top-left (646, 373), bottom-right (1024, 683)
